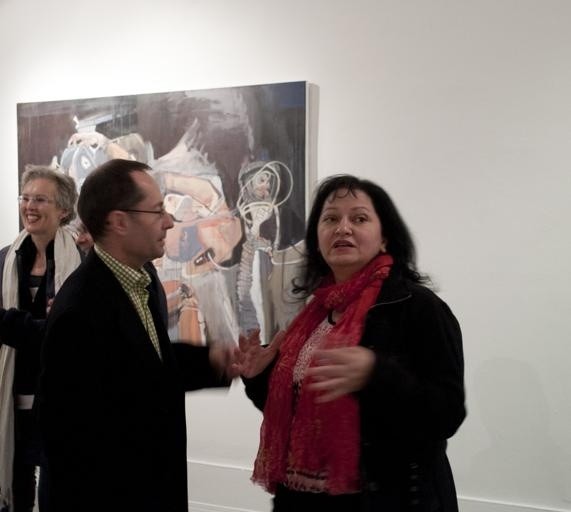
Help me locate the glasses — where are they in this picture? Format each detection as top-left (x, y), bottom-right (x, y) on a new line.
top-left (17, 194), bottom-right (58, 207)
top-left (104, 206), bottom-right (167, 219)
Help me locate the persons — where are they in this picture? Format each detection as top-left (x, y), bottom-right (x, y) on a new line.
top-left (35, 159), bottom-right (249, 510)
top-left (226, 170), bottom-right (469, 512)
top-left (46, 219), bottom-right (170, 332)
top-left (0, 163), bottom-right (85, 512)
